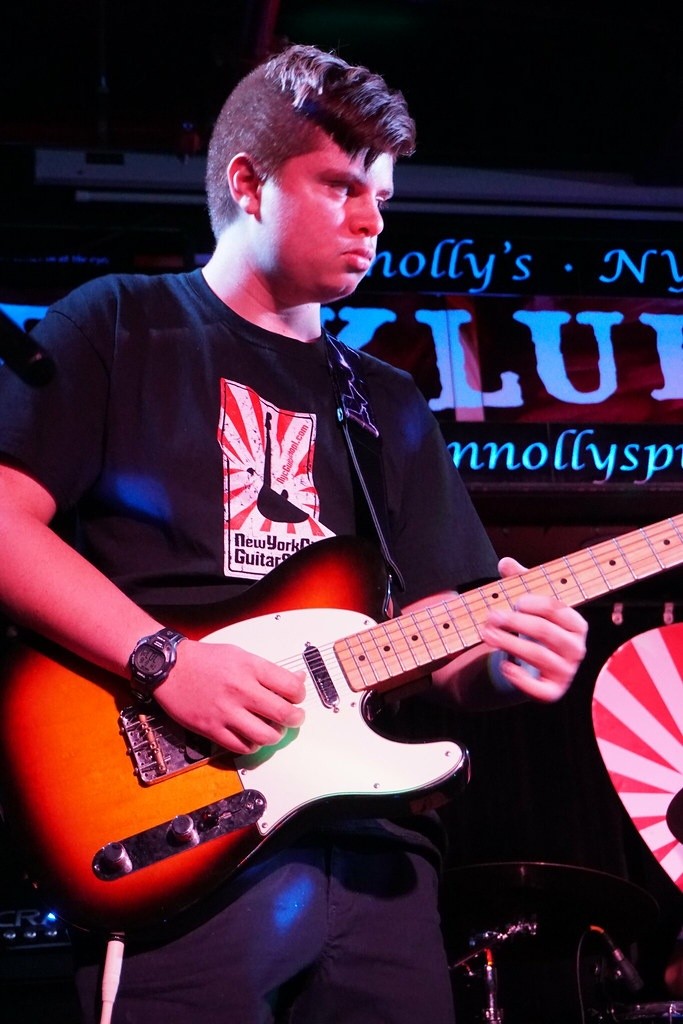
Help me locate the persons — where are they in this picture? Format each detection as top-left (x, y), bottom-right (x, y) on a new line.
top-left (0, 43), bottom-right (589, 1024)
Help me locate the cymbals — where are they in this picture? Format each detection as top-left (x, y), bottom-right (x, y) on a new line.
top-left (440, 860), bottom-right (664, 944)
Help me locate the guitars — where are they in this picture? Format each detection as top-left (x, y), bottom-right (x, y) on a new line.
top-left (0, 512), bottom-right (683, 947)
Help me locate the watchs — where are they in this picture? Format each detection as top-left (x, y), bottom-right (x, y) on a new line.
top-left (129, 627), bottom-right (187, 711)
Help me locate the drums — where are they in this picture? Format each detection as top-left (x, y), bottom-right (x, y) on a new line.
top-left (588, 1000), bottom-right (683, 1024)
